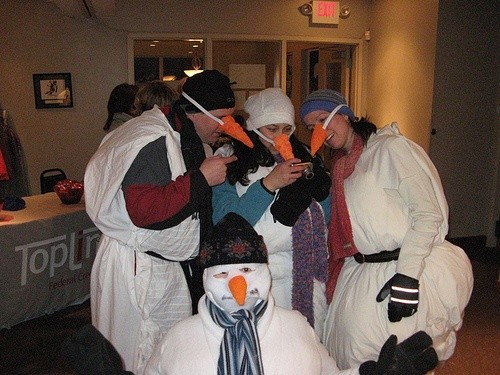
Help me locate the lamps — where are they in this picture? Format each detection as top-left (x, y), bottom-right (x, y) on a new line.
top-left (183, 44), bottom-right (204, 78)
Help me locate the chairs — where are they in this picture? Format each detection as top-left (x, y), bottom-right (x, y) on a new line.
top-left (40, 168), bottom-right (67, 195)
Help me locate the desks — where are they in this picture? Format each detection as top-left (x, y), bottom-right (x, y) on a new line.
top-left (0, 191), bottom-right (102, 330)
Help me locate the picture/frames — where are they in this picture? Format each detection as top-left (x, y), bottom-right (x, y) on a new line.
top-left (300, 47), bottom-right (318, 107)
top-left (32, 73), bottom-right (73, 110)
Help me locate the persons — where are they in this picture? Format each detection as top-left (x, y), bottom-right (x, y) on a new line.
top-left (212, 88), bottom-right (333, 343)
top-left (142, 212), bottom-right (439, 375)
top-left (134, 81), bottom-right (180, 115)
top-left (83, 69), bottom-right (254, 375)
top-left (103, 82), bottom-right (142, 133)
top-left (299, 88), bottom-right (474, 375)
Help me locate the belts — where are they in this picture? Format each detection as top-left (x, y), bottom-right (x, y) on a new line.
top-left (145, 251), bottom-right (191, 262)
top-left (354, 248), bottom-right (402, 264)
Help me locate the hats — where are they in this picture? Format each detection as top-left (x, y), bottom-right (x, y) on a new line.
top-left (177, 70), bottom-right (235, 113)
top-left (299, 89), bottom-right (355, 118)
top-left (244, 88), bottom-right (296, 131)
top-left (199, 212), bottom-right (268, 270)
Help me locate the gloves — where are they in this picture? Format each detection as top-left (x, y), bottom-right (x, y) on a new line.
top-left (62, 325), bottom-right (134, 375)
top-left (359, 330), bottom-right (439, 375)
top-left (377, 273), bottom-right (420, 322)
top-left (270, 176), bottom-right (312, 226)
top-left (310, 171), bottom-right (332, 202)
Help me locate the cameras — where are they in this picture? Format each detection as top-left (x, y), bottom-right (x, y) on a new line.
top-left (290, 162), bottom-right (314, 180)
